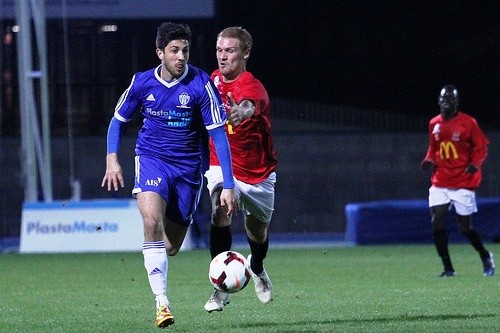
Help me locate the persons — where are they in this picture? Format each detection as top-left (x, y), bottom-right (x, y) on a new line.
top-left (204, 27), bottom-right (277, 313)
top-left (101, 22), bottom-right (241, 328)
top-left (421, 85), bottom-right (496, 277)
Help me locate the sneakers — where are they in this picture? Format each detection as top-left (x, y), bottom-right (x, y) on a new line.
top-left (154, 304), bottom-right (175, 328)
top-left (245, 253), bottom-right (274, 305)
top-left (203, 287), bottom-right (230, 314)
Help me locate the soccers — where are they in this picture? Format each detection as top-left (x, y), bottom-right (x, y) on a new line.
top-left (207, 250), bottom-right (251, 292)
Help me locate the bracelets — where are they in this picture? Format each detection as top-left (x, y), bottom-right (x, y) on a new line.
top-left (246, 109), bottom-right (254, 117)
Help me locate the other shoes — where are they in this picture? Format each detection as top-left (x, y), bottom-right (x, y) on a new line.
top-left (438, 269), bottom-right (456, 278)
top-left (481, 252), bottom-right (496, 278)
